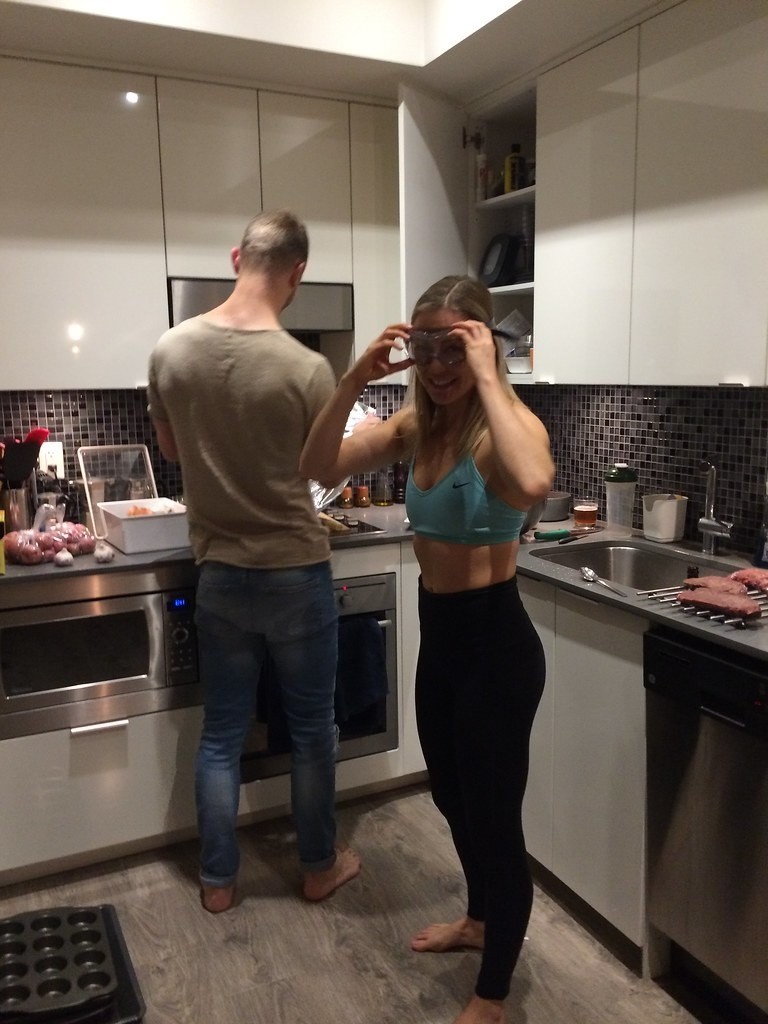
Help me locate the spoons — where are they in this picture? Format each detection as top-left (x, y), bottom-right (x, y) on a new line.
top-left (579, 567), bottom-right (628, 597)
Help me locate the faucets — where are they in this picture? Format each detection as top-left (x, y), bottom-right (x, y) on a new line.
top-left (694, 461), bottom-right (736, 556)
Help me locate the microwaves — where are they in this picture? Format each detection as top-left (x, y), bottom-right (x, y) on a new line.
top-left (0, 564), bottom-right (204, 741)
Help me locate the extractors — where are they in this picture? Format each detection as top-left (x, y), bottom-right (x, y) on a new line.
top-left (168, 278), bottom-right (353, 332)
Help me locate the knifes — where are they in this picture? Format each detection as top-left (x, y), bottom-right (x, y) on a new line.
top-left (558, 534), bottom-right (588, 544)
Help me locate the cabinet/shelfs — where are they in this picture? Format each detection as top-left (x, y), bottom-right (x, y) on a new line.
top-left (0, 0), bottom-right (767, 390)
top-left (0, 499), bottom-right (768, 980)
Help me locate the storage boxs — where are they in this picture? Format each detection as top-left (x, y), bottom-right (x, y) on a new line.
top-left (97, 497), bottom-right (191, 554)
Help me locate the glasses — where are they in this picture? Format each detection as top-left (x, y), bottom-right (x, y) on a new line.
top-left (406, 329), bottom-right (466, 366)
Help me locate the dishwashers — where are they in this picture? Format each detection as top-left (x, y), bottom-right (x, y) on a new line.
top-left (644, 630), bottom-right (768, 1012)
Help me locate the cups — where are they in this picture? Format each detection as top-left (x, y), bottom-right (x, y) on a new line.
top-left (68, 476), bottom-right (146, 524)
top-left (3, 489), bottom-right (32, 534)
top-left (574, 496), bottom-right (599, 530)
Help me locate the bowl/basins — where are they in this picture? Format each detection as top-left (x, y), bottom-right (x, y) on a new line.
top-left (503, 357), bottom-right (532, 373)
top-left (539, 492), bottom-right (571, 522)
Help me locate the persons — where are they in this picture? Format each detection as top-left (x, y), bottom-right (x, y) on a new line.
top-left (147, 213), bottom-right (384, 911)
top-left (299, 273), bottom-right (555, 1024)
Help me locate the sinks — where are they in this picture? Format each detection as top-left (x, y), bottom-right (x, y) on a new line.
top-left (528, 539), bottom-right (745, 593)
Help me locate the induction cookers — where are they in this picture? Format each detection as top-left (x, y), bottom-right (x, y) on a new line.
top-left (318, 509), bottom-right (385, 537)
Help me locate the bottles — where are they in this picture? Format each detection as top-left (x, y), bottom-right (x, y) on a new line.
top-left (395, 461), bottom-right (406, 503)
top-left (605, 463), bottom-right (638, 538)
top-left (505, 144), bottom-right (525, 193)
top-left (340, 487), bottom-right (354, 508)
top-left (752, 495), bottom-right (768, 568)
top-left (356, 485), bottom-right (370, 506)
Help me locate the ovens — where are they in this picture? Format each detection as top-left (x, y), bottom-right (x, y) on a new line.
top-left (239, 573), bottom-right (398, 788)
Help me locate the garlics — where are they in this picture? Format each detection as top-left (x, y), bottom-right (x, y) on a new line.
top-left (55, 548), bottom-right (74, 566)
top-left (94, 544), bottom-right (116, 562)
